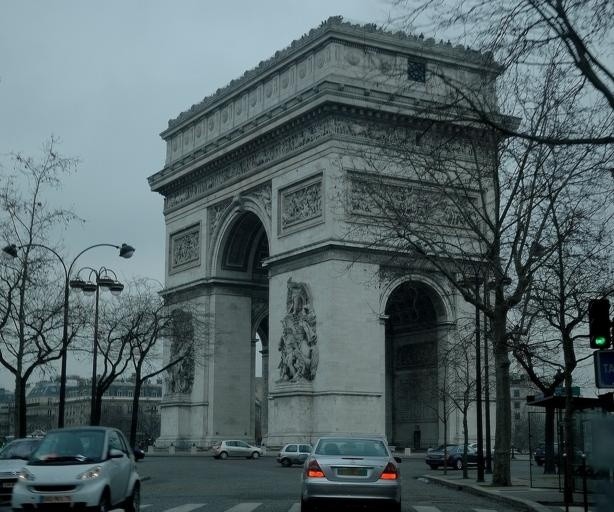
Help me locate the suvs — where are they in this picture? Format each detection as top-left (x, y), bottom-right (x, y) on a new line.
top-left (536, 442), bottom-right (584, 468)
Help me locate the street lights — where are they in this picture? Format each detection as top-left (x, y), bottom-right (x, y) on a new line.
top-left (7, 243), bottom-right (135, 432)
top-left (411, 235), bottom-right (546, 481)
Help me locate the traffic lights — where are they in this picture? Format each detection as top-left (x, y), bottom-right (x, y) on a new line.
top-left (588, 298), bottom-right (610, 349)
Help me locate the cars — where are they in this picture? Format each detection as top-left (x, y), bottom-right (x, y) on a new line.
top-left (216, 439), bottom-right (264, 460)
top-left (279, 440), bottom-right (319, 467)
top-left (301, 436), bottom-right (401, 511)
top-left (0, 427), bottom-right (140, 512)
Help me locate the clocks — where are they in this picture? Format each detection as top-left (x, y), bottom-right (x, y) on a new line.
top-left (408, 62), bottom-right (425, 83)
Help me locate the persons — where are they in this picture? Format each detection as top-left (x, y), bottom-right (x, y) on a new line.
top-left (136, 433), bottom-right (153, 451)
top-left (210, 205), bottom-right (228, 233)
top-left (352, 181), bottom-right (477, 228)
top-left (174, 233), bottom-right (199, 266)
top-left (0, 433), bottom-right (7, 449)
top-left (280, 186), bottom-right (321, 226)
top-left (251, 189), bottom-right (271, 215)
top-left (278, 279), bottom-right (317, 383)
